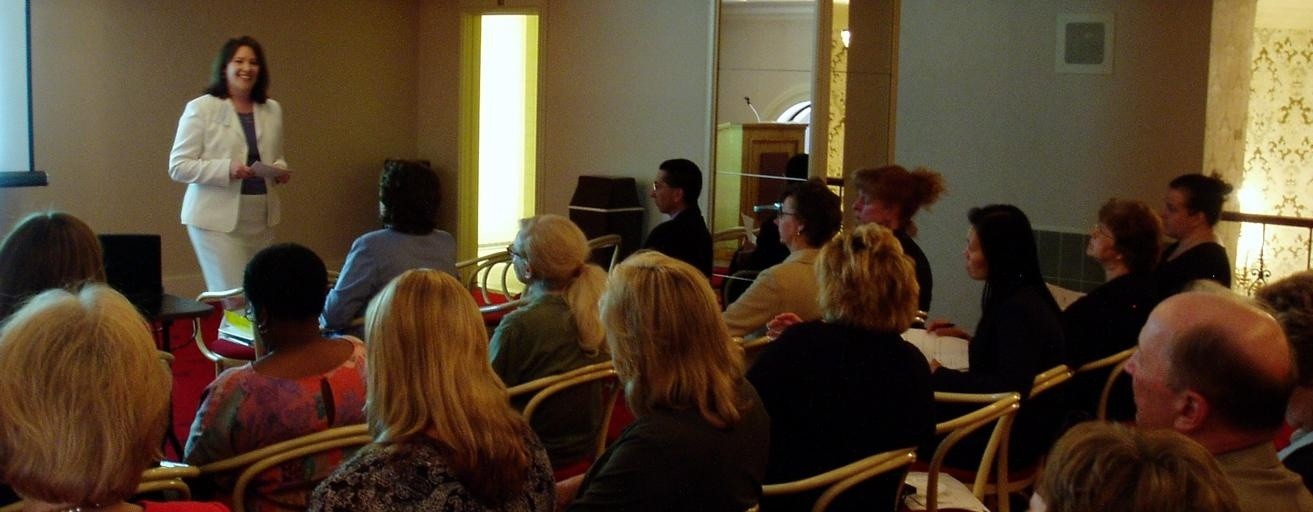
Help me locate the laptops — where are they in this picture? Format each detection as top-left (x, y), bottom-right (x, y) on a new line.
top-left (97, 232), bottom-right (215, 319)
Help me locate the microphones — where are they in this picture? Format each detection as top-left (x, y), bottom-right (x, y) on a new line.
top-left (742, 93), bottom-right (760, 124)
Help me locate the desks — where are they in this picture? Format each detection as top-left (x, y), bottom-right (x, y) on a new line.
top-left (124, 294), bottom-right (214, 460)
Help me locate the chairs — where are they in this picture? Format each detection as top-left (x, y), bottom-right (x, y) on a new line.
top-left (492, 361), bottom-right (619, 482)
top-left (1078, 347), bottom-right (1140, 422)
top-left (906, 365), bottom-right (1072, 511)
top-left (192, 270), bottom-right (340, 379)
top-left (732, 336), bottom-right (776, 371)
top-left (586, 234), bottom-right (622, 272)
top-left (900, 392), bottom-right (1020, 512)
top-left (455, 250), bottom-right (532, 327)
top-left (1, 466), bottom-right (199, 510)
top-left (198, 421), bottom-right (375, 509)
top-left (762, 447), bottom-right (916, 512)
top-left (711, 226), bottom-right (749, 289)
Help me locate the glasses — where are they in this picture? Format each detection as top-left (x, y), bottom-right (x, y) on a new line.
top-left (653, 183), bottom-right (679, 192)
top-left (778, 206), bottom-right (801, 220)
top-left (507, 244), bottom-right (524, 259)
top-left (1096, 223), bottom-right (1116, 242)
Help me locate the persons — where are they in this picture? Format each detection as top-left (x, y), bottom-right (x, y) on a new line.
top-left (487, 215), bottom-right (610, 470)
top-left (319, 162), bottom-right (457, 338)
top-left (1030, 417), bottom-right (1240, 512)
top-left (1062, 196), bottom-right (1167, 420)
top-left (851, 164), bottom-right (950, 329)
top-left (1160, 170), bottom-right (1232, 306)
top-left (925, 202), bottom-right (1082, 452)
top-left (740, 223), bottom-right (939, 511)
top-left (0, 212), bottom-right (104, 319)
top-left (1251, 268), bottom-right (1313, 492)
top-left (554, 249), bottom-right (775, 512)
top-left (720, 176), bottom-right (844, 345)
top-left (313, 268), bottom-right (554, 512)
top-left (1, 282), bottom-right (232, 512)
top-left (185, 244), bottom-right (371, 512)
top-left (643, 158), bottom-right (715, 283)
top-left (168, 35), bottom-right (290, 295)
top-left (1126, 290), bottom-right (1313, 511)
top-left (728, 150), bottom-right (808, 275)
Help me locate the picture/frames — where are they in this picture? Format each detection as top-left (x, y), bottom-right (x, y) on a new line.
top-left (1054, 11), bottom-right (1113, 75)
